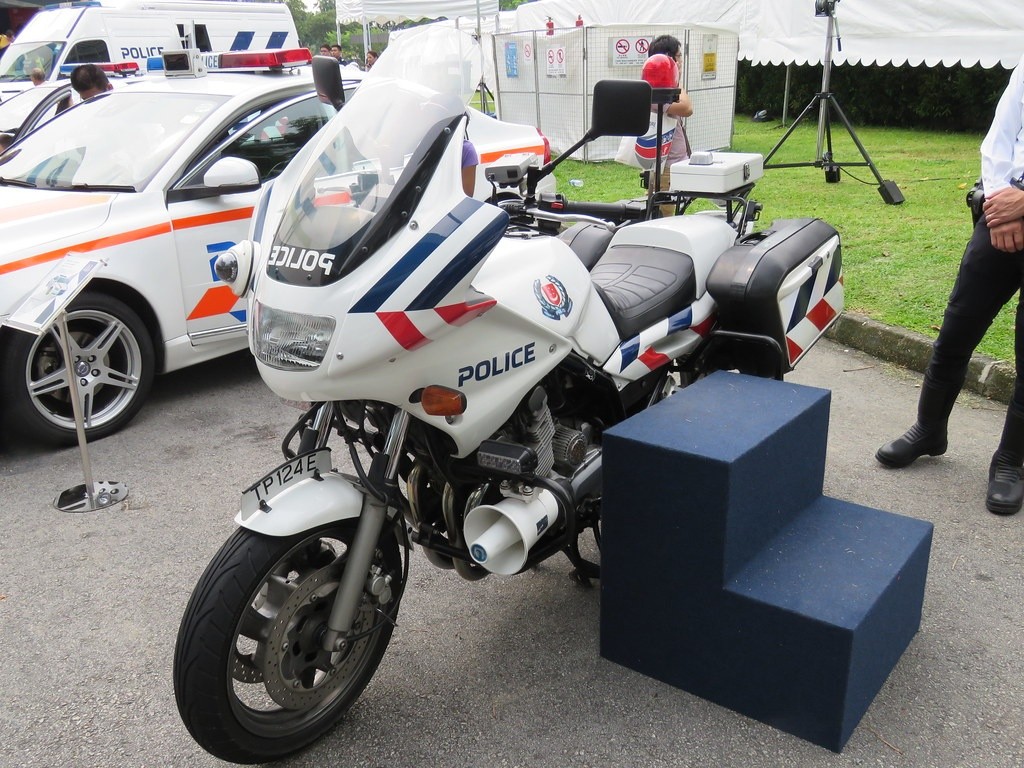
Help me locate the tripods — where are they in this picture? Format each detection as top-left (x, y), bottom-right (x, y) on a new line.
top-left (764, 0), bottom-right (905, 206)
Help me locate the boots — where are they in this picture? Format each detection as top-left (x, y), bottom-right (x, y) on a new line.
top-left (985, 394), bottom-right (1024, 516)
top-left (875, 375), bottom-right (965, 469)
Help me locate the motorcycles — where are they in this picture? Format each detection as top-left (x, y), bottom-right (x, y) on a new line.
top-left (170, 20), bottom-right (846, 767)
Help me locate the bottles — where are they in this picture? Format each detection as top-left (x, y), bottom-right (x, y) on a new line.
top-left (569, 180), bottom-right (584, 187)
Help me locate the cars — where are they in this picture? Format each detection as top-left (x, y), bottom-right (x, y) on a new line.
top-left (0, 46), bottom-right (561, 448)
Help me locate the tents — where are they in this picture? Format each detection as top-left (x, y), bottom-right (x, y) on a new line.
top-left (336, 1), bottom-right (500, 113)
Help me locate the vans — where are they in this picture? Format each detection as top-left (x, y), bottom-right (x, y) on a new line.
top-left (0, 1), bottom-right (303, 163)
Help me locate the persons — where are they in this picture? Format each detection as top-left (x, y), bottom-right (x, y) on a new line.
top-left (0, 30), bottom-right (14, 49)
top-left (875, 53), bottom-right (1024, 514)
top-left (320, 45), bottom-right (348, 66)
top-left (31, 68), bottom-right (45, 86)
top-left (424, 95), bottom-right (479, 198)
top-left (647, 34), bottom-right (694, 217)
top-left (57, 64), bottom-right (113, 108)
top-left (367, 51), bottom-right (379, 72)
